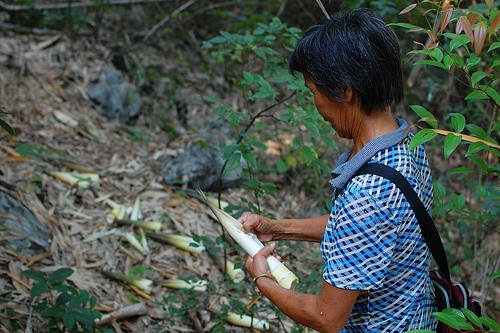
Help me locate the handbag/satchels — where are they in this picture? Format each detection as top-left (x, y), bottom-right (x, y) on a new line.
top-left (429, 268), bottom-right (491, 333)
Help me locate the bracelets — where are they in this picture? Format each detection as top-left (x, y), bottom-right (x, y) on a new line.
top-left (252, 274), bottom-right (278, 283)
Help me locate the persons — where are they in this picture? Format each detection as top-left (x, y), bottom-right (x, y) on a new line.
top-left (237, 8), bottom-right (438, 333)
